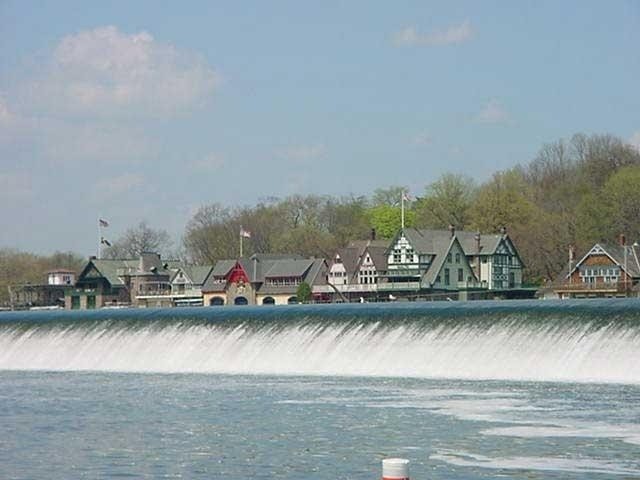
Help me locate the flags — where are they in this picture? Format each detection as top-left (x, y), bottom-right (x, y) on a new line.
top-left (240, 228), bottom-right (251, 238)
top-left (401, 193), bottom-right (414, 202)
top-left (99, 218), bottom-right (109, 228)
top-left (100, 237), bottom-right (112, 248)
